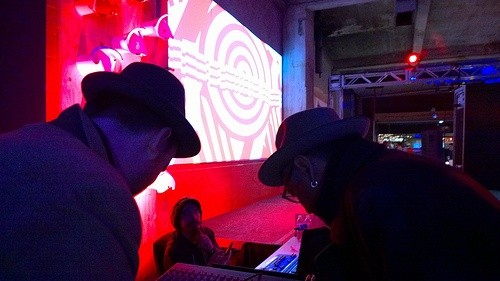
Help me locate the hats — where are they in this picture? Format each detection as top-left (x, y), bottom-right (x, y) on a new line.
top-left (258, 108), bottom-right (370, 185)
top-left (172, 199), bottom-right (202, 230)
top-left (80, 62), bottom-right (201, 159)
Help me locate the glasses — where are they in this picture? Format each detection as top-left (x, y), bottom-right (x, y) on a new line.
top-left (282, 166), bottom-right (302, 204)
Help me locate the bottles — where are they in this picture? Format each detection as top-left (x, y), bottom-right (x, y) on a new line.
top-left (296, 215), bottom-right (305, 241)
top-left (412, 134), bottom-right (421, 155)
top-left (305, 215), bottom-right (312, 229)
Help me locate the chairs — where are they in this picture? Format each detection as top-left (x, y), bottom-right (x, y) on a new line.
top-left (154, 227), bottom-right (221, 267)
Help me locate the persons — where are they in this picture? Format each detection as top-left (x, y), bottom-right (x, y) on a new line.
top-left (163, 198), bottom-right (232, 266)
top-left (0, 61), bottom-right (202, 281)
top-left (258, 107), bottom-right (500, 281)
